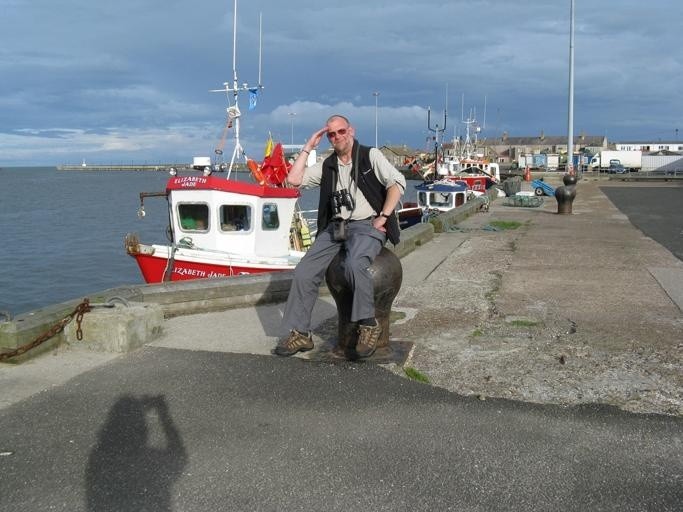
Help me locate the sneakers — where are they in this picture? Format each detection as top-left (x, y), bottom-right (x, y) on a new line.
top-left (355, 317), bottom-right (382, 356)
top-left (275, 329), bottom-right (314, 355)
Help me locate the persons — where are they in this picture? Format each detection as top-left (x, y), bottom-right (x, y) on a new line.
top-left (274, 114), bottom-right (407, 359)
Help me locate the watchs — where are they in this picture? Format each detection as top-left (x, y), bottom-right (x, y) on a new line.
top-left (380, 211), bottom-right (390, 219)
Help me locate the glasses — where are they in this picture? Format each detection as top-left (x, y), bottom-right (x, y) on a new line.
top-left (327, 127), bottom-right (349, 138)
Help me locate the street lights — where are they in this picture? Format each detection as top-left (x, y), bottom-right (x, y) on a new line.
top-left (370, 89), bottom-right (380, 146)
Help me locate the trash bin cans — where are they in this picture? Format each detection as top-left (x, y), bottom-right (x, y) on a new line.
top-left (503, 176), bottom-right (523, 197)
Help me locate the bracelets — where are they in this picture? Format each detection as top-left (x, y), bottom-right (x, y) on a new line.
top-left (302, 149), bottom-right (310, 155)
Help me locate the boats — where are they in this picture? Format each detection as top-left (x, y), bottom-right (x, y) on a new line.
top-left (401, 82), bottom-right (499, 214)
top-left (126, 0), bottom-right (305, 283)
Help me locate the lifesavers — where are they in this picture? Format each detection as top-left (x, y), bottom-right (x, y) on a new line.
top-left (247, 160), bottom-right (266, 184)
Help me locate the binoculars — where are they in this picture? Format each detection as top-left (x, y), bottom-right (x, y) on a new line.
top-left (329, 188), bottom-right (355, 213)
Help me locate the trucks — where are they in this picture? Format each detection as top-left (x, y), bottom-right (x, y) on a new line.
top-left (588, 151), bottom-right (641, 172)
top-left (511, 154), bottom-right (560, 170)
